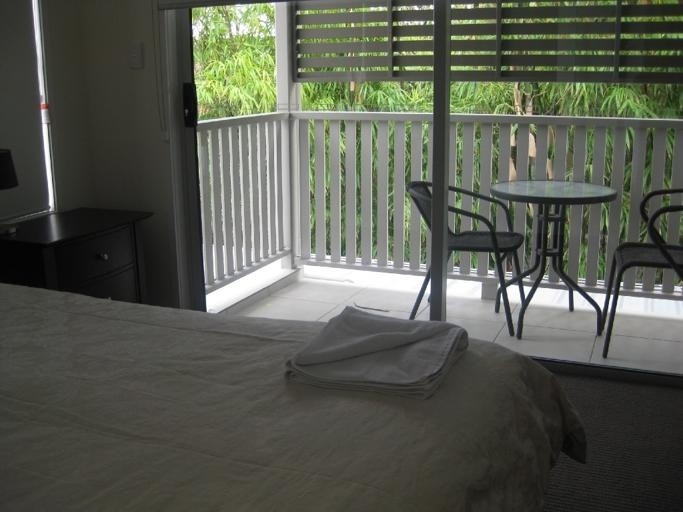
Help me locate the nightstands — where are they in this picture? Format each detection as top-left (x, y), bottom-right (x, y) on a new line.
top-left (0, 204), bottom-right (158, 305)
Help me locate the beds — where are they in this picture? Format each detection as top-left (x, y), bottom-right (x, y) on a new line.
top-left (0, 280), bottom-right (588, 512)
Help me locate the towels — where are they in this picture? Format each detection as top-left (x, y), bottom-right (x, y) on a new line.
top-left (282, 303), bottom-right (469, 402)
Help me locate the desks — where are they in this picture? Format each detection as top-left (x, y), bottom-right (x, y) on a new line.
top-left (488, 176), bottom-right (616, 339)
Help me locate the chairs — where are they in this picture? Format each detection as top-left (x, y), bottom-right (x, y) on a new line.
top-left (405, 180), bottom-right (525, 335)
top-left (597, 187), bottom-right (683, 360)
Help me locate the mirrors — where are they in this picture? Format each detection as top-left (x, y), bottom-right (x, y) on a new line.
top-left (0, 0), bottom-right (62, 227)
